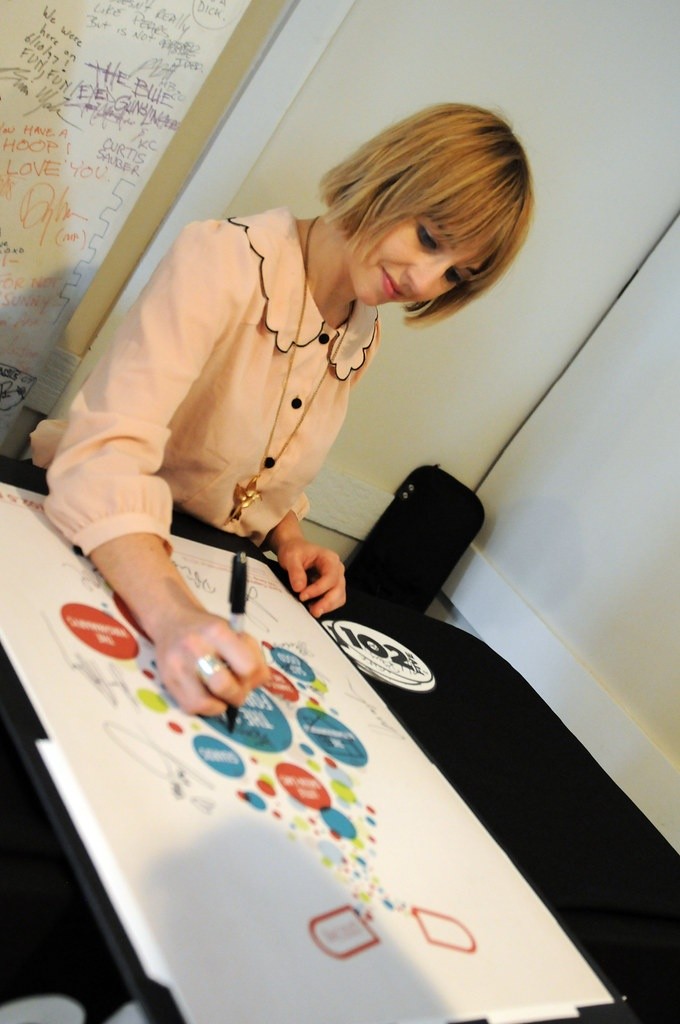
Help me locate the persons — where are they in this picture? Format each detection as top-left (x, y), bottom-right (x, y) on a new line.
top-left (3, 103), bottom-right (535, 719)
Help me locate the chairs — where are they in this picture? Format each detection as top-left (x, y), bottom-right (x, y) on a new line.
top-left (346, 465), bottom-right (486, 616)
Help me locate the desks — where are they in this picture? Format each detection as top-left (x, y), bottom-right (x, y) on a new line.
top-left (0, 456), bottom-right (680, 1023)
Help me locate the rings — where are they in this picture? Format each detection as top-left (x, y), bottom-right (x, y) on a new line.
top-left (195, 655), bottom-right (227, 683)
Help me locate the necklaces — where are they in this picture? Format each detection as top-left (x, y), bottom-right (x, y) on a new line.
top-left (221, 215), bottom-right (349, 523)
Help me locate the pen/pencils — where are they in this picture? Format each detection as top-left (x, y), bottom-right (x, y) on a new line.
top-left (224, 552), bottom-right (248, 736)
